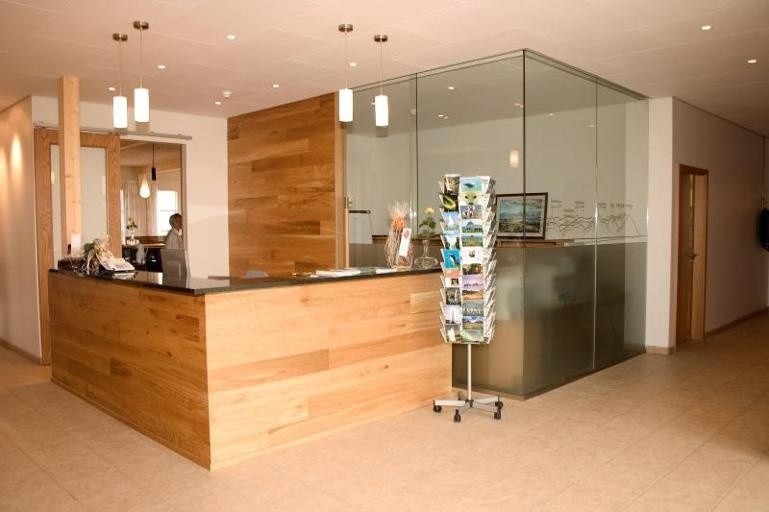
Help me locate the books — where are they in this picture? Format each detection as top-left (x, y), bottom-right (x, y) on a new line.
top-left (438, 174), bottom-right (498, 345)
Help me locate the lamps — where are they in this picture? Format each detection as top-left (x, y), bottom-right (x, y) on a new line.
top-left (337, 21), bottom-right (354, 123)
top-left (374, 34), bottom-right (393, 127)
top-left (139, 144), bottom-right (151, 200)
top-left (109, 17), bottom-right (156, 131)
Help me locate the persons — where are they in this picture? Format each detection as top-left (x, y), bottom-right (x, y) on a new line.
top-left (164, 212), bottom-right (184, 248)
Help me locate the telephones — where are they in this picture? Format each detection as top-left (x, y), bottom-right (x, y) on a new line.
top-left (100, 256), bottom-right (135, 273)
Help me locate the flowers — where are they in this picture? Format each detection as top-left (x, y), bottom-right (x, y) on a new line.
top-left (421, 208), bottom-right (436, 235)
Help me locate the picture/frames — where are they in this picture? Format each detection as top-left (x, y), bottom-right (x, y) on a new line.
top-left (494, 193), bottom-right (547, 240)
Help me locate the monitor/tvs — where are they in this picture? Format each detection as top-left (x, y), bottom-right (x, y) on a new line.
top-left (160, 248), bottom-right (190, 277)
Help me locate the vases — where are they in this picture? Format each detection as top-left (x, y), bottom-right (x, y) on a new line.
top-left (414, 238), bottom-right (439, 270)
